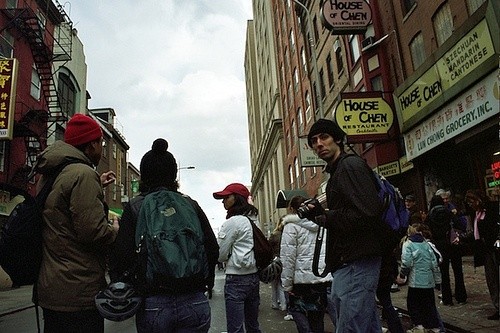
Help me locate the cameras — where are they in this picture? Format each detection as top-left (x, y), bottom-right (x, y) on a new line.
top-left (297, 199), bottom-right (321, 220)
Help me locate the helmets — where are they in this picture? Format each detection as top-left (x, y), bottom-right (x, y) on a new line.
top-left (94, 283), bottom-right (143, 322)
top-left (256, 256), bottom-right (282, 284)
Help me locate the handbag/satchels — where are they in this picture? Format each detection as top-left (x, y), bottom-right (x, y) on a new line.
top-left (0, 198), bottom-right (40, 286)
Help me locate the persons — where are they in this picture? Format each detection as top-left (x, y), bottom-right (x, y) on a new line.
top-left (465, 189), bottom-right (500, 320)
top-left (306, 119), bottom-right (404, 333)
top-left (397, 223), bottom-right (447, 333)
top-left (280, 196), bottom-right (334, 333)
top-left (109, 150), bottom-right (219, 333)
top-left (213, 183), bottom-right (262, 333)
top-left (405, 195), bottom-right (416, 211)
top-left (267, 222), bottom-right (293, 321)
top-left (422, 189), bottom-right (468, 304)
top-left (33, 114), bottom-right (119, 333)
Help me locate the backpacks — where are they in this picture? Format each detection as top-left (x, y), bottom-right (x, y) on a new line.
top-left (246, 217), bottom-right (274, 270)
top-left (431, 206), bottom-right (454, 242)
top-left (340, 154), bottom-right (409, 234)
top-left (133, 188), bottom-right (209, 289)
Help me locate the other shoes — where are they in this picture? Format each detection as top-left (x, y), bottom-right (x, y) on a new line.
top-left (487, 312), bottom-right (500, 320)
top-left (406, 327), bottom-right (427, 333)
top-left (271, 304), bottom-right (286, 312)
top-left (284, 315), bottom-right (293, 320)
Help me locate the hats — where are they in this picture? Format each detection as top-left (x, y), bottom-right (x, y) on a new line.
top-left (307, 119), bottom-right (345, 148)
top-left (64, 114), bottom-right (102, 146)
top-left (405, 195), bottom-right (417, 203)
top-left (139, 139), bottom-right (176, 184)
top-left (212, 183), bottom-right (248, 199)
